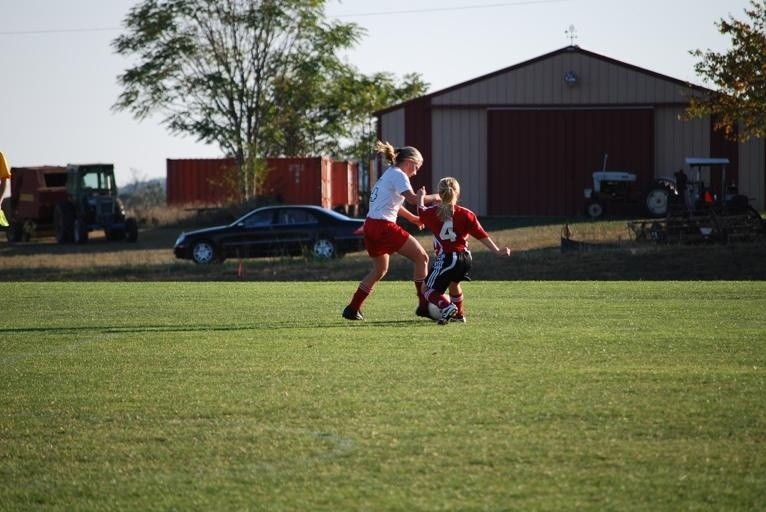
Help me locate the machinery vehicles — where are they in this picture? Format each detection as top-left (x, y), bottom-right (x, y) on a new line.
top-left (583, 167), bottom-right (689, 219)
top-left (2, 163), bottom-right (138, 245)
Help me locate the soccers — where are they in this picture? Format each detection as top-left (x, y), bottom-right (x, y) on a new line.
top-left (428, 294), bottom-right (451, 320)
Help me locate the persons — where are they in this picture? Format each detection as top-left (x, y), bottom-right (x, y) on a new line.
top-left (0, 151), bottom-right (12, 210)
top-left (416, 176), bottom-right (511, 327)
top-left (674, 168), bottom-right (689, 194)
top-left (340, 139), bottom-right (436, 321)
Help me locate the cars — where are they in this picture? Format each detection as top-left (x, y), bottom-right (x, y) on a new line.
top-left (172, 203), bottom-right (365, 266)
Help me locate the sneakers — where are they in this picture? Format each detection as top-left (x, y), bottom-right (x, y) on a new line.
top-left (437, 304), bottom-right (465, 325)
top-left (415, 304), bottom-right (435, 320)
top-left (342, 305), bottom-right (363, 320)
